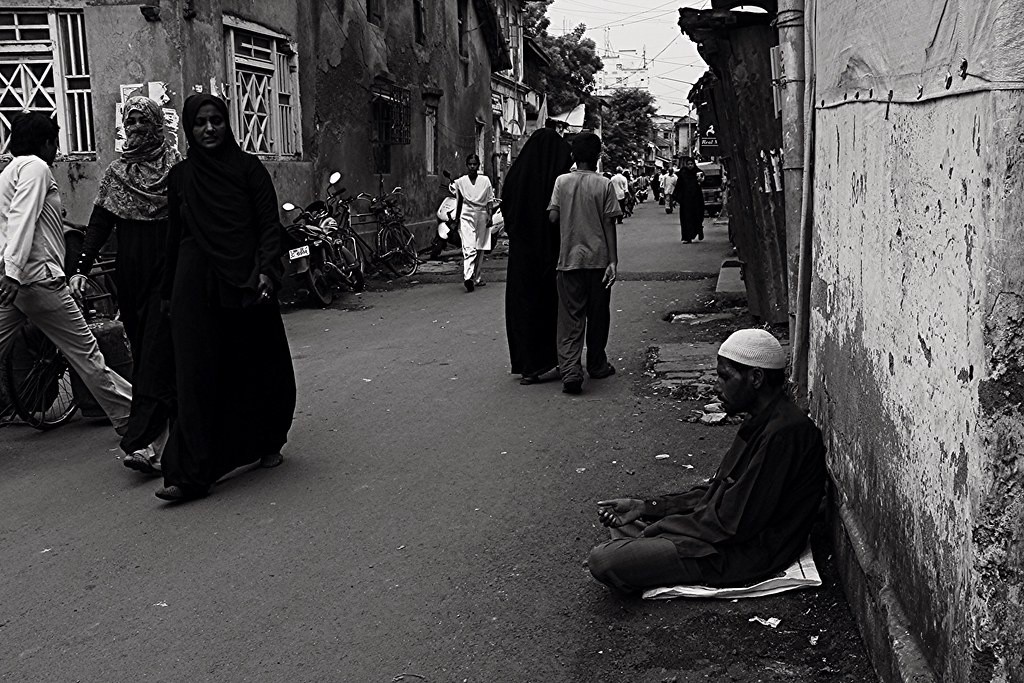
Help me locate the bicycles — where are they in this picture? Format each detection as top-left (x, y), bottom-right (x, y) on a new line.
top-left (354, 185), bottom-right (428, 277)
top-left (0, 220), bottom-right (127, 432)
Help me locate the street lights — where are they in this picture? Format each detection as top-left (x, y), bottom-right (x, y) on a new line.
top-left (687, 100), bottom-right (709, 160)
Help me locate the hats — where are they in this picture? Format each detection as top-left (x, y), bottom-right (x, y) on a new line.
top-left (717, 329), bottom-right (785, 369)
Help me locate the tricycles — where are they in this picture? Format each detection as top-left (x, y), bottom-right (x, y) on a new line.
top-left (695, 162), bottom-right (725, 218)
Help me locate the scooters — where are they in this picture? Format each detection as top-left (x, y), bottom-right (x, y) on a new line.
top-left (637, 185), bottom-right (649, 202)
top-left (429, 169), bottom-right (506, 259)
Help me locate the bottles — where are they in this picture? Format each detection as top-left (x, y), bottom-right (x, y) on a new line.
top-left (70, 311), bottom-right (133, 417)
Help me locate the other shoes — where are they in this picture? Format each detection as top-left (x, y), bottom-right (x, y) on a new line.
top-left (465, 279), bottom-right (474, 292)
top-left (589, 363), bottom-right (616, 379)
top-left (123, 451), bottom-right (161, 475)
top-left (156, 487), bottom-right (184, 502)
top-left (520, 375), bottom-right (541, 384)
top-left (563, 379), bottom-right (583, 394)
top-left (260, 449), bottom-right (284, 468)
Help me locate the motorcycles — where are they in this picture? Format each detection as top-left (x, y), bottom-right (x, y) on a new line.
top-left (280, 171), bottom-right (365, 307)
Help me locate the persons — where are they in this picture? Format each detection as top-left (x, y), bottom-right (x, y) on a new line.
top-left (67, 94), bottom-right (186, 478)
top-left (153, 93), bottom-right (297, 503)
top-left (499, 127), bottom-right (573, 386)
top-left (453, 153), bottom-right (494, 292)
top-left (0, 112), bottom-right (133, 437)
top-left (586, 328), bottom-right (826, 602)
top-left (671, 158), bottom-right (707, 244)
top-left (602, 165), bottom-right (679, 225)
top-left (546, 132), bottom-right (624, 395)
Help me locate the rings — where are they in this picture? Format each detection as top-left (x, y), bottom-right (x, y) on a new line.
top-left (262, 292), bottom-right (271, 300)
